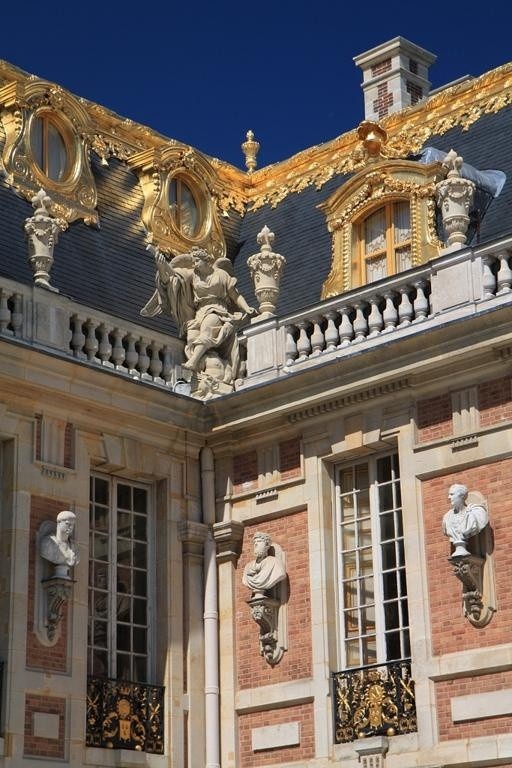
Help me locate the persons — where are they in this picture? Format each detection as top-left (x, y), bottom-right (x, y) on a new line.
top-left (94, 567), bottom-right (131, 637)
top-left (40, 510), bottom-right (81, 567)
top-left (441, 484), bottom-right (489, 542)
top-left (154, 246), bottom-right (260, 373)
top-left (242, 532), bottom-right (287, 592)
